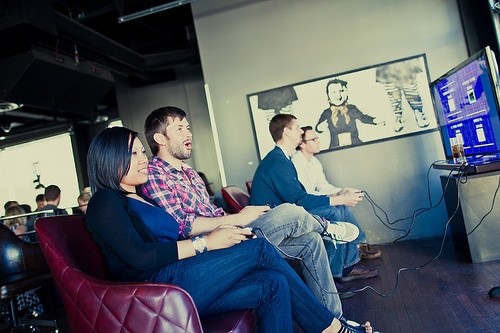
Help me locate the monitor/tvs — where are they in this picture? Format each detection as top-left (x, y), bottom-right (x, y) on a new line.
top-left (429, 46), bottom-right (500, 165)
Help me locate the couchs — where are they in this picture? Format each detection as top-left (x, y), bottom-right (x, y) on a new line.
top-left (34, 204), bottom-right (259, 333)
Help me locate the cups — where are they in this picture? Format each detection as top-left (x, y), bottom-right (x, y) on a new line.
top-left (449, 137), bottom-right (467, 164)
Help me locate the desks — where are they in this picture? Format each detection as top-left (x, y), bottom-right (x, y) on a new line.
top-left (433, 159), bottom-right (500, 265)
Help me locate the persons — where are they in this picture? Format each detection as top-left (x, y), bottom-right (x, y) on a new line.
top-left (290, 126), bottom-right (382, 261)
top-left (315, 80), bottom-right (385, 150)
top-left (375, 56), bottom-right (429, 132)
top-left (136, 106), bottom-right (363, 329)
top-left (0, 185), bottom-right (91, 243)
top-left (249, 114), bottom-right (379, 299)
top-left (84, 126), bottom-right (377, 333)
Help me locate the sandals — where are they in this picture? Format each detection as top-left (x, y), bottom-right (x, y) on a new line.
top-left (336, 313), bottom-right (376, 333)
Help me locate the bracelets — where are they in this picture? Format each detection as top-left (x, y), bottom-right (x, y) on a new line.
top-left (190, 234), bottom-right (208, 257)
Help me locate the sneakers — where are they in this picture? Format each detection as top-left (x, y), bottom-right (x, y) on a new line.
top-left (394, 115), bottom-right (404, 131)
top-left (320, 217), bottom-right (360, 245)
top-left (414, 110), bottom-right (429, 127)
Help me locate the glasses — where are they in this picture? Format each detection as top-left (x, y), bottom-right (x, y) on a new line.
top-left (305, 136), bottom-right (319, 142)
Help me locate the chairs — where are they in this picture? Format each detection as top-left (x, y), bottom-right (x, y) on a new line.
top-left (222, 186), bottom-right (251, 214)
top-left (0, 222), bottom-right (60, 333)
top-left (246, 181), bottom-right (251, 195)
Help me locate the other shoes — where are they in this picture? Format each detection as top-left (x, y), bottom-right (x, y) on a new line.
top-left (340, 265), bottom-right (377, 281)
top-left (360, 243), bottom-right (382, 258)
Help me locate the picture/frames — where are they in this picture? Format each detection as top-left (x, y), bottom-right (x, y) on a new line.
top-left (246, 52), bottom-right (440, 161)
